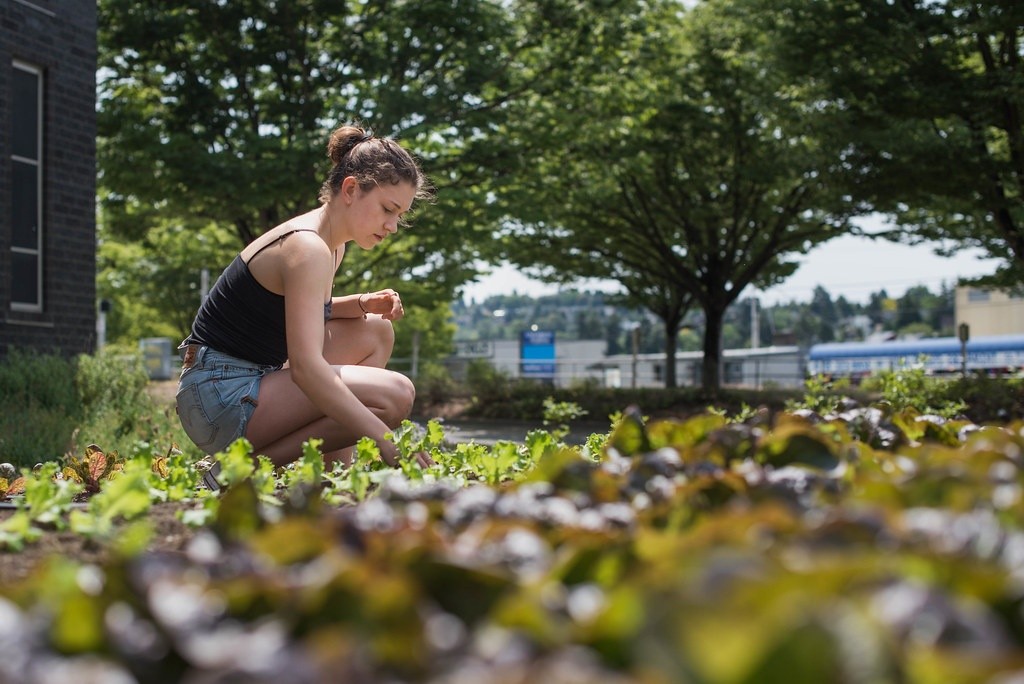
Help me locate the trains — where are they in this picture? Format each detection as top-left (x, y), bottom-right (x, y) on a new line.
top-left (589, 334), bottom-right (1024, 384)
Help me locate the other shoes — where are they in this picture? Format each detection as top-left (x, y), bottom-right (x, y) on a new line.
top-left (202, 462), bottom-right (223, 491)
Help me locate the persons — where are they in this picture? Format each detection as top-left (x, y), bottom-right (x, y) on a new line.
top-left (175, 126), bottom-right (439, 493)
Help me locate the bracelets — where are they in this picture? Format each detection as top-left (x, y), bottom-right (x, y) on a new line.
top-left (357, 294), bottom-right (369, 319)
